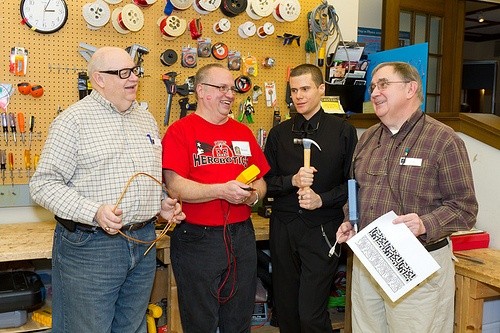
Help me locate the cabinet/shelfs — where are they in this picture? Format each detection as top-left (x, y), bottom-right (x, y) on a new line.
top-left (323, 53), bottom-right (369, 115)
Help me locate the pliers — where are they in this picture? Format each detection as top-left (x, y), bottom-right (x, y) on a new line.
top-left (237, 96), bottom-right (255, 124)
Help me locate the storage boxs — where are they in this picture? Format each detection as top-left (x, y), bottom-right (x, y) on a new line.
top-left (451, 233), bottom-right (490, 251)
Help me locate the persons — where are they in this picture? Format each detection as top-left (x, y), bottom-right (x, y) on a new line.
top-left (264, 64), bottom-right (358, 333)
top-left (161, 64), bottom-right (271, 333)
top-left (30, 47), bottom-right (186, 333)
top-left (336, 62), bottom-right (478, 333)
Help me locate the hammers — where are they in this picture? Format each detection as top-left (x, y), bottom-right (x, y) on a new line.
top-left (294, 138), bottom-right (322, 191)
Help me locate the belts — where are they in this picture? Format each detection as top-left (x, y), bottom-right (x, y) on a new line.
top-left (55, 213), bottom-right (158, 232)
top-left (423, 237), bottom-right (449, 252)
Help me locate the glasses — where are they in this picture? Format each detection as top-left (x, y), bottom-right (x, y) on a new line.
top-left (201, 82), bottom-right (237, 94)
top-left (99, 64), bottom-right (141, 79)
top-left (367, 79), bottom-right (411, 94)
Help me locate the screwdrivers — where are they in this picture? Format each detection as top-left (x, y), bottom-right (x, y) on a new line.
top-left (18, 112), bottom-right (25, 144)
top-left (34, 155), bottom-right (38, 170)
top-left (8, 152), bottom-right (14, 187)
top-left (9, 113), bottom-right (16, 143)
top-left (24, 149), bottom-right (30, 180)
top-left (0, 150), bottom-right (6, 185)
top-left (29, 115), bottom-right (35, 148)
top-left (2, 112), bottom-right (8, 144)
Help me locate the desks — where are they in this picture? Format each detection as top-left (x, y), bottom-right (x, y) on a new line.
top-left (252, 207), bottom-right (351, 333)
top-left (0, 222), bottom-right (183, 333)
top-left (452, 247), bottom-right (500, 333)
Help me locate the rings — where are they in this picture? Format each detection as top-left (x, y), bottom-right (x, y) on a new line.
top-left (301, 195), bottom-right (303, 199)
top-left (242, 197), bottom-right (247, 201)
top-left (105, 227), bottom-right (110, 232)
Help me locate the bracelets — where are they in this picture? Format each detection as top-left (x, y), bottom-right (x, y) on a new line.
top-left (247, 192), bottom-right (259, 206)
